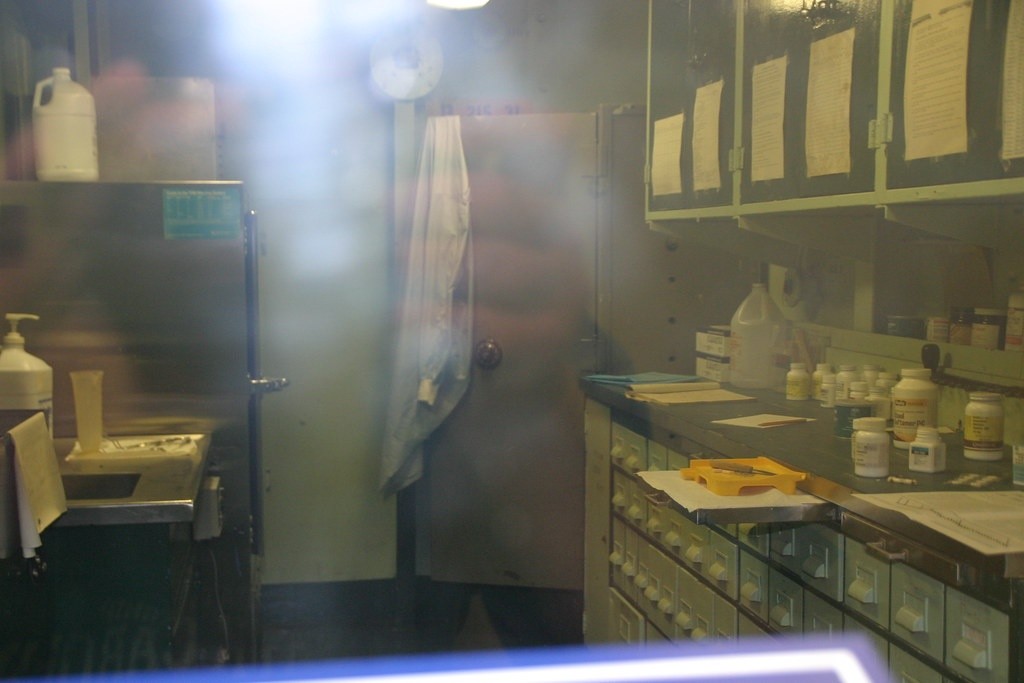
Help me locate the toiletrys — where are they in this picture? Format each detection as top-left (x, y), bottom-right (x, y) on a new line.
top-left (0, 313), bottom-right (53, 438)
top-left (68, 370), bottom-right (105, 453)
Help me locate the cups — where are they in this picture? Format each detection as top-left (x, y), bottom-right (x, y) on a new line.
top-left (71, 371), bottom-right (103, 455)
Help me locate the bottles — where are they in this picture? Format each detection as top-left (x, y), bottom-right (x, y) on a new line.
top-left (786, 363), bottom-right (810, 400)
top-left (887, 308), bottom-right (1007, 350)
top-left (891, 387), bottom-right (894, 400)
top-left (835, 365), bottom-right (859, 399)
top-left (963, 392), bottom-right (1004, 461)
top-left (855, 418), bottom-right (889, 477)
top-left (909, 427), bottom-right (946, 472)
top-left (875, 372), bottom-right (897, 394)
top-left (811, 363), bottom-right (834, 400)
top-left (820, 376), bottom-right (835, 408)
top-left (893, 368), bottom-right (938, 450)
top-left (728, 277), bottom-right (784, 390)
top-left (865, 385), bottom-right (891, 423)
top-left (861, 364), bottom-right (881, 386)
top-left (1004, 291), bottom-right (1024, 353)
top-left (851, 420), bottom-right (855, 460)
top-left (848, 382), bottom-right (867, 399)
top-left (31, 67), bottom-right (99, 182)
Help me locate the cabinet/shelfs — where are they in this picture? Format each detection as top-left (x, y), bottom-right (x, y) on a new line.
top-left (583, 400), bottom-right (1024, 683)
top-left (644, 0), bottom-right (1024, 263)
